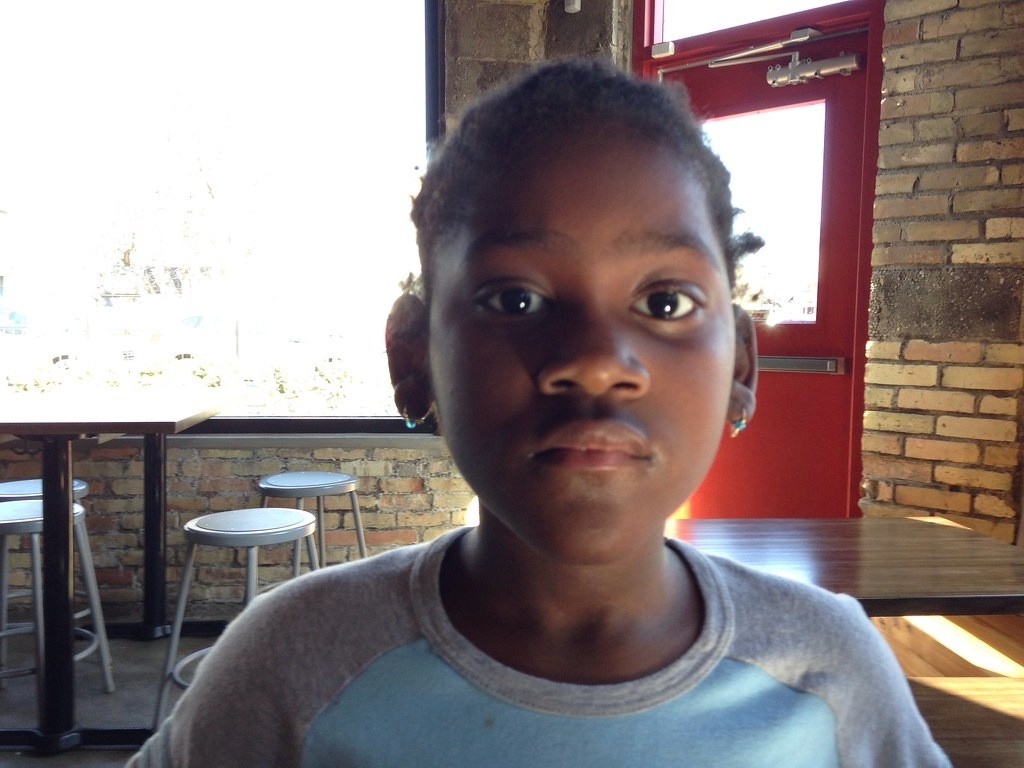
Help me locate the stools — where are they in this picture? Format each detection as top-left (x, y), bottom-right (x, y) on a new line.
top-left (151, 507), bottom-right (323, 739)
top-left (0, 499), bottom-right (116, 694)
top-left (0, 478), bottom-right (115, 671)
top-left (242, 470), bottom-right (369, 615)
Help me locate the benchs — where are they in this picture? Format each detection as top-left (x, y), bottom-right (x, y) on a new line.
top-left (905, 676), bottom-right (1024, 768)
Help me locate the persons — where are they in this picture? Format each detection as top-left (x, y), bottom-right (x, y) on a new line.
top-left (126, 53), bottom-right (955, 768)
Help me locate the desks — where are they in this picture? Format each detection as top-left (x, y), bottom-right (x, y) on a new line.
top-left (660, 515), bottom-right (1024, 619)
top-left (0, 405), bottom-right (226, 754)
